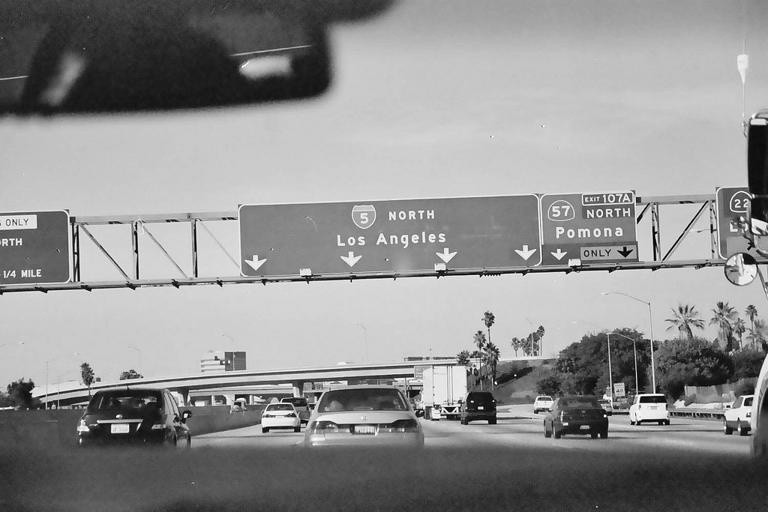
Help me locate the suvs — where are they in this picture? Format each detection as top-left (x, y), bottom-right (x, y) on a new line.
top-left (461, 392), bottom-right (497, 425)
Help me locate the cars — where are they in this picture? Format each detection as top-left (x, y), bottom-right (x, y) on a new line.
top-left (78, 389), bottom-right (191, 448)
top-left (722, 394), bottom-right (754, 436)
top-left (262, 386), bottom-right (425, 448)
top-left (543, 396), bottom-right (608, 438)
top-left (534, 396), bottom-right (555, 414)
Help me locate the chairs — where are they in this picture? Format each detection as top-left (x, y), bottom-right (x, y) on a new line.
top-left (378, 401), bottom-right (395, 410)
top-left (147, 402), bottom-right (157, 407)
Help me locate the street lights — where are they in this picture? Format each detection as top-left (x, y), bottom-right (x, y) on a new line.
top-left (605, 292), bottom-right (656, 394)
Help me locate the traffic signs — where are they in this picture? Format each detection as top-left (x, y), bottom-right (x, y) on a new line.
top-left (237, 193), bottom-right (541, 278)
top-left (540, 191), bottom-right (639, 269)
top-left (715, 187), bottom-right (768, 262)
top-left (0, 209), bottom-right (72, 286)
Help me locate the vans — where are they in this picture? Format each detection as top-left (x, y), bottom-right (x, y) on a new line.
top-left (629, 393), bottom-right (670, 425)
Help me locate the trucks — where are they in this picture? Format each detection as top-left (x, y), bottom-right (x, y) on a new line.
top-left (420, 366), bottom-right (468, 421)
